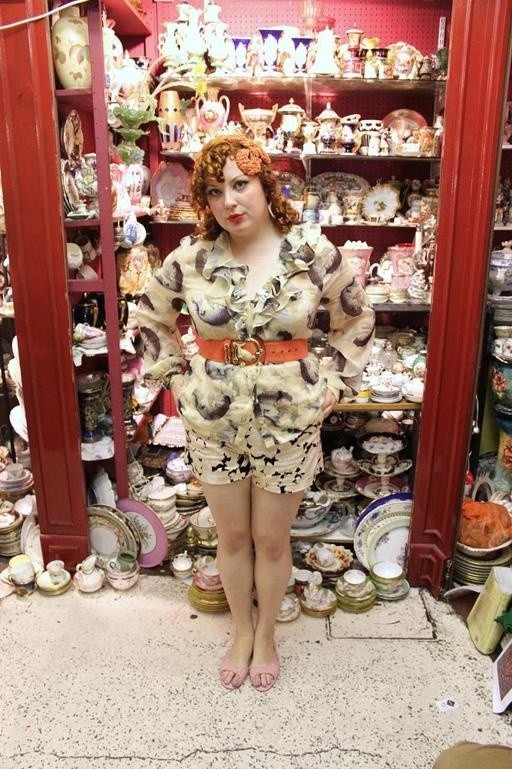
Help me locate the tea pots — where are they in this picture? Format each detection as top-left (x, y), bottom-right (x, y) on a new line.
top-left (336, 241), bottom-right (433, 305)
top-left (105, 550), bottom-right (140, 590)
top-left (155, 86), bottom-right (442, 157)
top-left (154, 2), bottom-right (446, 80)
top-left (73, 555), bottom-right (105, 592)
top-left (151, 163), bottom-right (438, 227)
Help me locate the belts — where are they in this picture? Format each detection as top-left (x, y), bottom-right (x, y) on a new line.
top-left (195, 335), bottom-right (308, 365)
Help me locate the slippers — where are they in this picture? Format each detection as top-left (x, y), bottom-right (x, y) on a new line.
top-left (248, 638), bottom-right (280, 693)
top-left (218, 648), bottom-right (249, 690)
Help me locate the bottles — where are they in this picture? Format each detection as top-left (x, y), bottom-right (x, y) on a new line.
top-left (389, 275), bottom-right (408, 303)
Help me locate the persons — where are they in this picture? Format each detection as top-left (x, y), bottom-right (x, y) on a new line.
top-left (137, 136), bottom-right (375, 692)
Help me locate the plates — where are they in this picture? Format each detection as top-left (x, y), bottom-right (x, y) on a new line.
top-left (36, 570), bottom-right (72, 596)
top-left (70, 292), bottom-right (108, 349)
top-left (60, 108), bottom-right (150, 220)
top-left (1, 205), bottom-right (44, 597)
top-left (150, 565), bottom-right (411, 622)
top-left (320, 412), bottom-right (416, 459)
top-left (82, 460), bottom-right (413, 566)
top-left (445, 450), bottom-right (511, 593)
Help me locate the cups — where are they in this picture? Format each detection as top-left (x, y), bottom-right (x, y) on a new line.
top-left (65, 227), bottom-right (102, 281)
top-left (343, 326), bottom-right (427, 406)
top-left (45, 560), bottom-right (65, 584)
